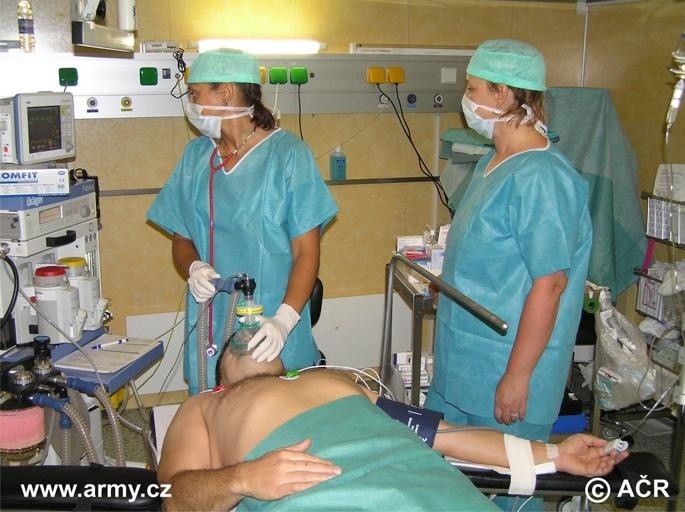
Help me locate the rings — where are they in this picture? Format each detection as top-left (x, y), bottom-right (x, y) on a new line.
top-left (510, 413), bottom-right (520, 418)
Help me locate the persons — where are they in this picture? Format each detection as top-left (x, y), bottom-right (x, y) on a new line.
top-left (143, 46), bottom-right (340, 396)
top-left (155, 326), bottom-right (630, 512)
top-left (418, 38), bottom-right (596, 512)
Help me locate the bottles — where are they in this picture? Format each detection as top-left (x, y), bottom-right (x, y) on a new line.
top-left (329, 145), bottom-right (346, 180)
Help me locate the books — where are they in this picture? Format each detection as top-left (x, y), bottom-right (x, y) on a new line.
top-left (52, 333), bottom-right (160, 375)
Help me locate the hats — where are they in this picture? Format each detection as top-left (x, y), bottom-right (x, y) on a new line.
top-left (464, 37), bottom-right (549, 93)
top-left (186, 47), bottom-right (262, 86)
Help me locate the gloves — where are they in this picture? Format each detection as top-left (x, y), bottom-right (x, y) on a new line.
top-left (247, 302), bottom-right (301, 363)
top-left (187, 260), bottom-right (221, 304)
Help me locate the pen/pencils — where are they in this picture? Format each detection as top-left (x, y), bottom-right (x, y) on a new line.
top-left (91, 338), bottom-right (129, 351)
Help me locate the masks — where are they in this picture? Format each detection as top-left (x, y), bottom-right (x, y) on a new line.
top-left (184, 102), bottom-right (223, 141)
top-left (460, 94), bottom-right (496, 140)
